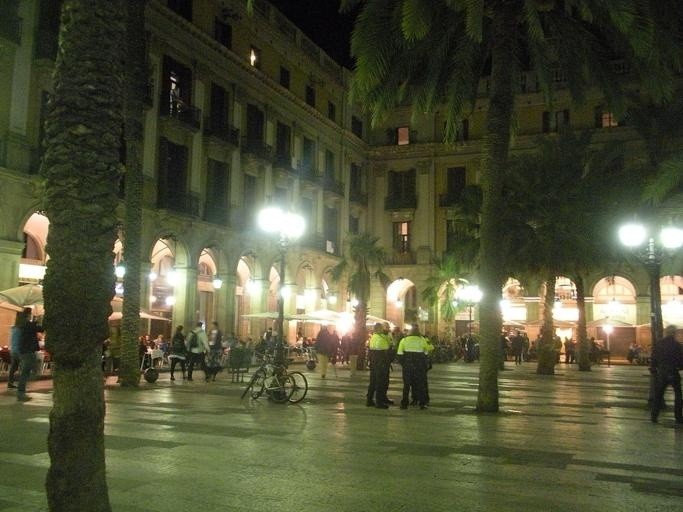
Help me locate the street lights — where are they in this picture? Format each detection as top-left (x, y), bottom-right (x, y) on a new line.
top-left (458, 285), bottom-right (483, 336)
top-left (619, 224), bottom-right (682, 346)
top-left (602, 323), bottom-right (613, 366)
top-left (260, 208), bottom-right (305, 348)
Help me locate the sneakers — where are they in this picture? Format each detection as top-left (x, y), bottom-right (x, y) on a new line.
top-left (366, 400), bottom-right (428, 410)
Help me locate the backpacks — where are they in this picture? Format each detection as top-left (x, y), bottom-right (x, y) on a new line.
top-left (190, 330), bottom-right (202, 347)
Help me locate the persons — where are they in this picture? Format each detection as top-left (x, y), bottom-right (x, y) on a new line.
top-left (8, 308), bottom-right (44, 402)
top-left (502, 331), bottom-right (599, 364)
top-left (169, 320), bottom-right (222, 381)
top-left (366, 322), bottom-right (435, 409)
top-left (296, 325), bottom-right (351, 379)
top-left (139, 334), bottom-right (167, 368)
top-left (263, 327), bottom-right (277, 359)
top-left (650, 326), bottom-right (682, 424)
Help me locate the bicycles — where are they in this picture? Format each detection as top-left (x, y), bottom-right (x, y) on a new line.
top-left (241, 351), bottom-right (308, 404)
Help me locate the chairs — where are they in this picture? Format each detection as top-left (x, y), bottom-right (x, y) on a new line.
top-left (0, 344), bottom-right (345, 375)
